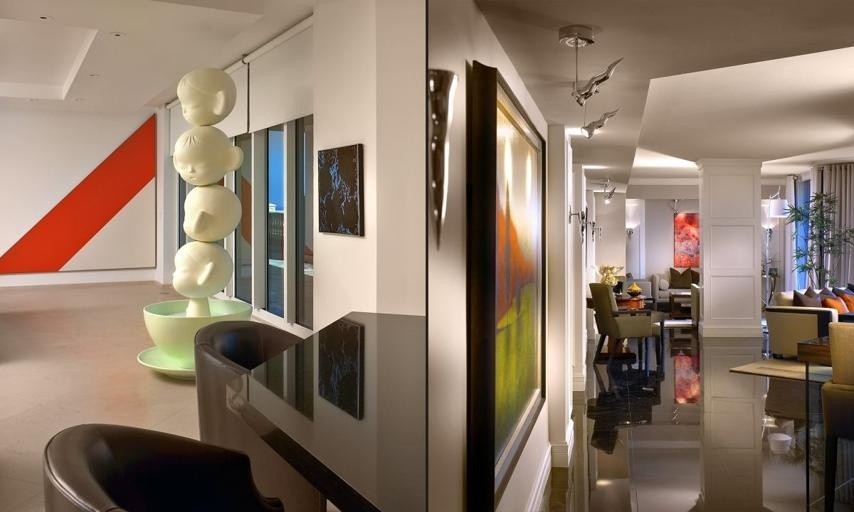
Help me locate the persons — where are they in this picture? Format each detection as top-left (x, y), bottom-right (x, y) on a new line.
top-left (170, 65), bottom-right (245, 299)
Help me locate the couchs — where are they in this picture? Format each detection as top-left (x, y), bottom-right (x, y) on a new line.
top-left (820, 322), bottom-right (853, 385)
top-left (689, 283), bottom-right (699, 326)
top-left (651, 270), bottom-right (699, 303)
top-left (763, 287), bottom-right (853, 359)
top-left (764, 377), bottom-right (826, 429)
top-left (192, 318), bottom-right (328, 511)
top-left (41, 422), bottom-right (286, 511)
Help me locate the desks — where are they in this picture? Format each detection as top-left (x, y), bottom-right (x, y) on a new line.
top-left (585, 295), bottom-right (655, 359)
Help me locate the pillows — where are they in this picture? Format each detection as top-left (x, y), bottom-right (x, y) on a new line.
top-left (668, 267), bottom-right (693, 289)
top-left (792, 282), bottom-right (853, 315)
top-left (659, 279), bottom-right (669, 291)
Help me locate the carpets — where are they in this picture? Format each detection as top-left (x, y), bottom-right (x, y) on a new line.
top-left (728, 356), bottom-right (831, 384)
top-left (652, 318), bottom-right (766, 330)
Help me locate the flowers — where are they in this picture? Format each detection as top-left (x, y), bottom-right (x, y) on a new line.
top-left (597, 264), bottom-right (624, 286)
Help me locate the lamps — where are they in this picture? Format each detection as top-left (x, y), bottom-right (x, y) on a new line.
top-left (669, 199), bottom-right (679, 214)
top-left (624, 228), bottom-right (633, 238)
top-left (768, 185), bottom-right (791, 219)
top-left (581, 217), bottom-right (601, 242)
top-left (599, 175), bottom-right (616, 202)
top-left (556, 25), bottom-right (625, 138)
top-left (760, 198), bottom-right (778, 334)
top-left (570, 208), bottom-right (586, 223)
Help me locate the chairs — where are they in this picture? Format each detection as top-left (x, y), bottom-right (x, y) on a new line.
top-left (589, 283), bottom-right (652, 366)
top-left (618, 337), bottom-right (665, 407)
top-left (621, 280), bottom-right (665, 350)
top-left (587, 364), bottom-right (652, 453)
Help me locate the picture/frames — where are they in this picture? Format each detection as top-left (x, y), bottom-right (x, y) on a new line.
top-left (463, 58), bottom-right (547, 509)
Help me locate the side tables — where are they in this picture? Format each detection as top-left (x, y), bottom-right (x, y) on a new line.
top-left (798, 335), bottom-right (832, 381)
